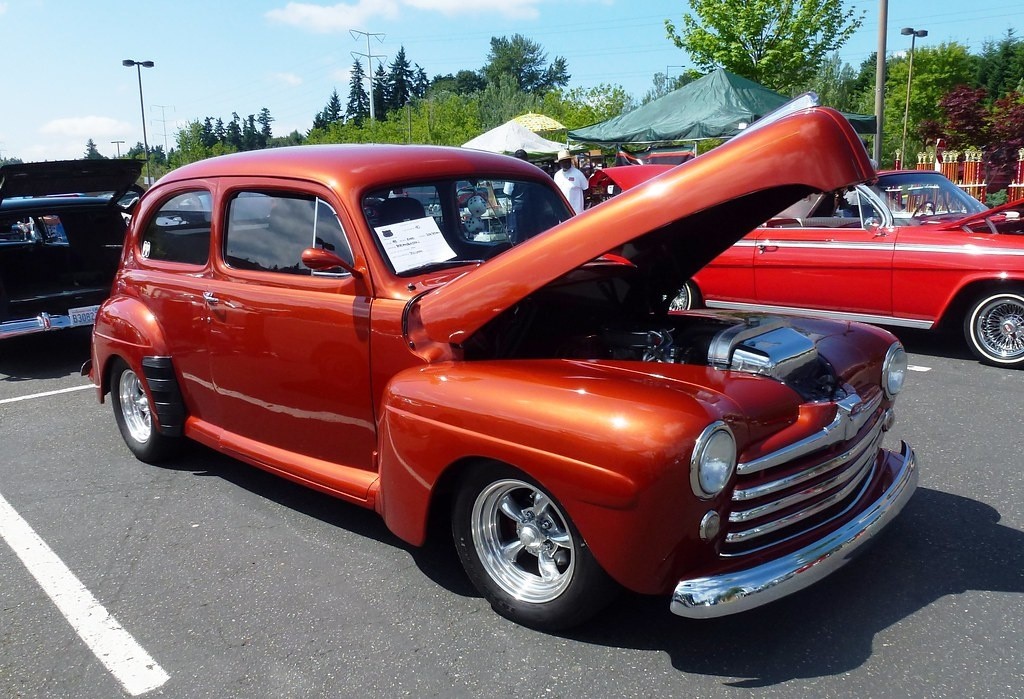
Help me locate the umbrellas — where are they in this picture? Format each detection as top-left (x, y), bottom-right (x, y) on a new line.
top-left (513, 110), bottom-right (567, 132)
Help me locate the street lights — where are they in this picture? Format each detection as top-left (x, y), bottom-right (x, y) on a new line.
top-left (121, 59), bottom-right (156, 189)
top-left (900, 26), bottom-right (928, 173)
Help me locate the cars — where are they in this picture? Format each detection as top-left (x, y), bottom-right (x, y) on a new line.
top-left (0, 156), bottom-right (150, 346)
top-left (587, 164), bottom-right (1024, 368)
top-left (80, 90), bottom-right (921, 631)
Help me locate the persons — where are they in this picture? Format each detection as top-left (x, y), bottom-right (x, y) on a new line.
top-left (503, 149), bottom-right (548, 243)
top-left (554, 149), bottom-right (588, 225)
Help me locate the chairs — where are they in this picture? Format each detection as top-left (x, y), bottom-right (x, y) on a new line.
top-left (376, 196), bottom-right (427, 228)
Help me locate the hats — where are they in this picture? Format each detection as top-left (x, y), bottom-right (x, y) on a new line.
top-left (553, 150), bottom-right (576, 164)
top-left (514, 149), bottom-right (527, 158)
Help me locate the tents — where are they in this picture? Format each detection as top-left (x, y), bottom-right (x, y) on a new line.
top-left (569, 65), bottom-right (877, 159)
top-left (461, 120), bottom-right (582, 157)
